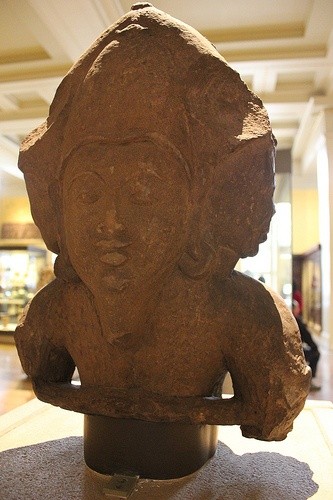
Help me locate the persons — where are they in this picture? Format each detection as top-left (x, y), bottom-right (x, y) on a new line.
top-left (292, 279), bottom-right (304, 321)
top-left (292, 300), bottom-right (322, 390)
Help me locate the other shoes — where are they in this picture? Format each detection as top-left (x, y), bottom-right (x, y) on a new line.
top-left (310, 383), bottom-right (321, 391)
